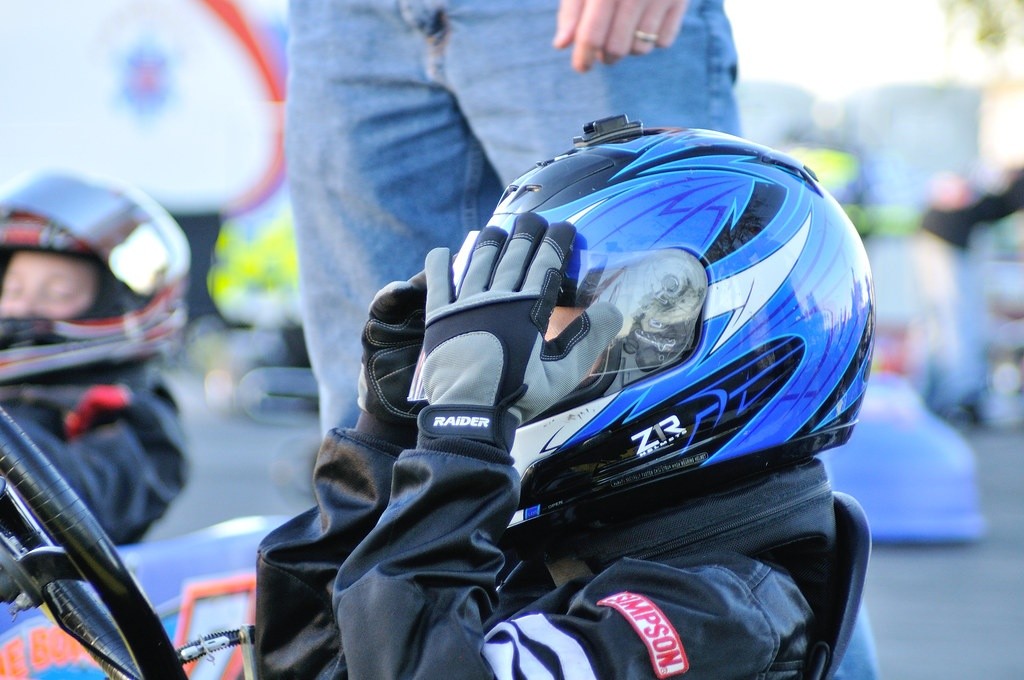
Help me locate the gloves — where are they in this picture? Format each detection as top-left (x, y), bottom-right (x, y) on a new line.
top-left (419, 210), bottom-right (623, 468)
top-left (355, 249), bottom-right (467, 448)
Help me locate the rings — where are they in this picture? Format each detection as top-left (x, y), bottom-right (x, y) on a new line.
top-left (637, 30), bottom-right (660, 43)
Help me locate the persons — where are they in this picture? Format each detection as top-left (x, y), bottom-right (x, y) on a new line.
top-left (287, 1), bottom-right (878, 679)
top-left (1, 172), bottom-right (193, 604)
top-left (251, 116), bottom-right (876, 680)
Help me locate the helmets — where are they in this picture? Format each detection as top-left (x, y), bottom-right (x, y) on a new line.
top-left (0, 170), bottom-right (192, 387)
top-left (442, 112), bottom-right (877, 531)
